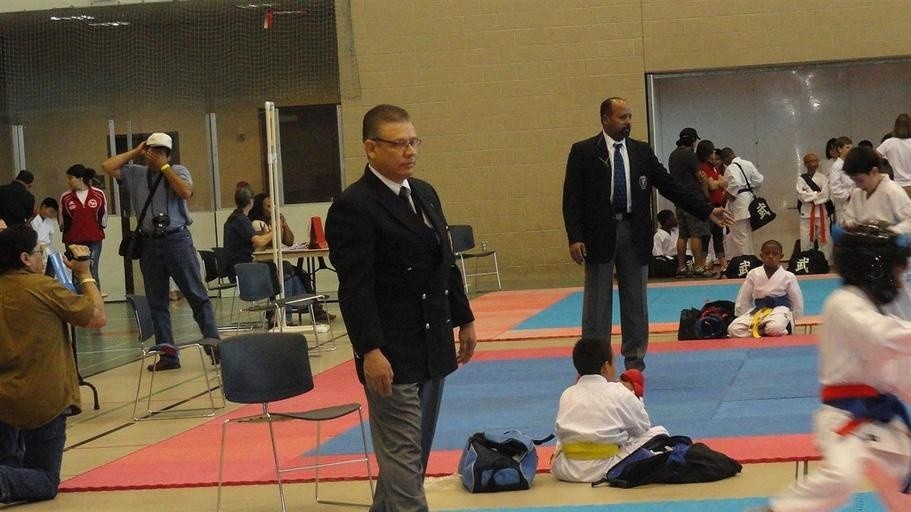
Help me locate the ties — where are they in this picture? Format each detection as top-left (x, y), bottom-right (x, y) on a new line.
top-left (399, 186), bottom-right (415, 214)
top-left (613, 143), bottom-right (627, 214)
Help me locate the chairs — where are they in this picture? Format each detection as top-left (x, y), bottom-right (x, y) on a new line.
top-left (195, 245), bottom-right (339, 357)
top-left (125, 293), bottom-right (226, 419)
top-left (448, 224), bottom-right (502, 293)
top-left (218, 331), bottom-right (376, 511)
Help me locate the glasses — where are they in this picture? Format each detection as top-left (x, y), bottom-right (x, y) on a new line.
top-left (371, 138), bottom-right (422, 149)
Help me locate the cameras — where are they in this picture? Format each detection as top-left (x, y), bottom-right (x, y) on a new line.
top-left (62, 247), bottom-right (73, 261)
top-left (152, 215), bottom-right (170, 235)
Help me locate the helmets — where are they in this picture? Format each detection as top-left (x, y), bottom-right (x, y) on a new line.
top-left (833, 218), bottom-right (911, 303)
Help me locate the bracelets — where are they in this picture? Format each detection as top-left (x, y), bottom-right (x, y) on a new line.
top-left (161, 164), bottom-right (170, 172)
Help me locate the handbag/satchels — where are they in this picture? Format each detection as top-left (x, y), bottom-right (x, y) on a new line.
top-left (307, 216), bottom-right (326, 248)
top-left (459, 429), bottom-right (538, 493)
top-left (119, 230), bottom-right (142, 259)
top-left (607, 434), bottom-right (743, 488)
top-left (727, 254), bottom-right (763, 278)
top-left (787, 251), bottom-right (829, 275)
top-left (748, 197), bottom-right (776, 231)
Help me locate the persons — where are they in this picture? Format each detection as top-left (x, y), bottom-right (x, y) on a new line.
top-left (563, 96), bottom-right (738, 377)
top-left (224, 182), bottom-right (337, 331)
top-left (770, 221), bottom-right (911, 512)
top-left (726, 240), bottom-right (804, 337)
top-left (100, 132), bottom-right (224, 372)
top-left (651, 127), bottom-right (764, 279)
top-left (551, 334), bottom-right (673, 482)
top-left (3, 164), bottom-right (108, 297)
top-left (795, 114), bottom-right (910, 321)
top-left (1, 227), bottom-right (107, 504)
top-left (322, 103), bottom-right (478, 512)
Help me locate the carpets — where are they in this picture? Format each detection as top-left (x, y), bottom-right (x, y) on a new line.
top-left (452, 270), bottom-right (841, 344)
top-left (60, 332), bottom-right (826, 484)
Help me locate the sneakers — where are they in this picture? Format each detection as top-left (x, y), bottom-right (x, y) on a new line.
top-left (315, 311), bottom-right (336, 320)
top-left (676, 263), bottom-right (727, 278)
top-left (204, 337), bottom-right (220, 355)
top-left (148, 360), bottom-right (179, 370)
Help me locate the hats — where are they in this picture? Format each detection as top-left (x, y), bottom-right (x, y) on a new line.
top-left (146, 133), bottom-right (172, 150)
top-left (680, 128), bottom-right (701, 140)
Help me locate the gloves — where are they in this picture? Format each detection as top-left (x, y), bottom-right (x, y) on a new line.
top-left (620, 369), bottom-right (644, 396)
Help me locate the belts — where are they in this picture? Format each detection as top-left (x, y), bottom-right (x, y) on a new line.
top-left (616, 212), bottom-right (631, 221)
top-left (141, 225), bottom-right (185, 238)
